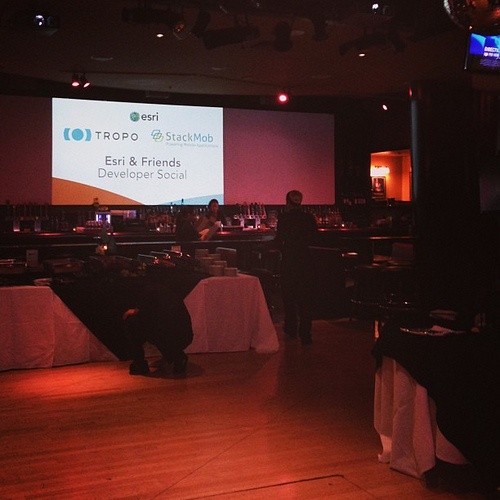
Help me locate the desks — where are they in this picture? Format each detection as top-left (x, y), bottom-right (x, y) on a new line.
top-left (371, 302), bottom-right (500, 482)
top-left (0, 272), bottom-right (280, 372)
top-left (353, 257), bottom-right (433, 317)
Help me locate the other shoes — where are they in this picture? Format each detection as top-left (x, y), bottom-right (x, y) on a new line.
top-left (300, 336), bottom-right (314, 343)
top-left (163, 352), bottom-right (188, 374)
top-left (130, 361), bottom-right (150, 374)
top-left (283, 326), bottom-right (297, 336)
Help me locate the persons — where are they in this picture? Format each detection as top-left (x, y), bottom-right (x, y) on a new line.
top-left (276, 190), bottom-right (319, 346)
top-left (118, 264), bottom-right (194, 375)
top-left (174, 207), bottom-right (219, 241)
top-left (206, 199), bottom-right (227, 232)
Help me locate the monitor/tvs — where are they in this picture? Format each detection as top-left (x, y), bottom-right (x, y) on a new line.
top-left (462, 31), bottom-right (500, 76)
top-left (94, 212), bottom-right (111, 225)
